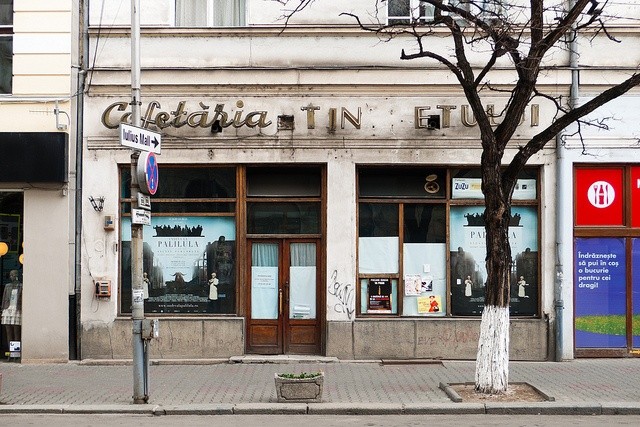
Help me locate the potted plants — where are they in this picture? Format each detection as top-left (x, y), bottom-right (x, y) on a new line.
top-left (274, 371), bottom-right (324, 404)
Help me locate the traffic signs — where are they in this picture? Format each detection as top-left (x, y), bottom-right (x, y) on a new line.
top-left (120, 122), bottom-right (161, 155)
top-left (137, 151), bottom-right (158, 195)
